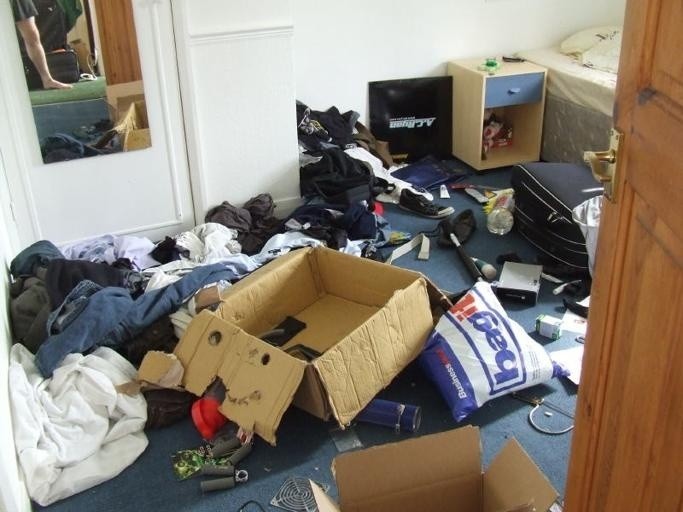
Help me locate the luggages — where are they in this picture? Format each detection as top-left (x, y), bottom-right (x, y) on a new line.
top-left (509, 159), bottom-right (611, 272)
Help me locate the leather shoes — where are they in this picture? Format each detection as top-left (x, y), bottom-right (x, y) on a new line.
top-left (435, 208), bottom-right (478, 248)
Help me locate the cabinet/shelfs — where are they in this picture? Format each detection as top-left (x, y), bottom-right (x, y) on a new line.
top-left (446, 56), bottom-right (547, 172)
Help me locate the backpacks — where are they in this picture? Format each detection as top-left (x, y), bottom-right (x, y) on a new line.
top-left (311, 146), bottom-right (396, 211)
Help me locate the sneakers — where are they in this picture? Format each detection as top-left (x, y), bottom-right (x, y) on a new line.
top-left (397, 188), bottom-right (455, 218)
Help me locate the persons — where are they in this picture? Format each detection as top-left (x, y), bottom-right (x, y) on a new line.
top-left (9, 0), bottom-right (75, 91)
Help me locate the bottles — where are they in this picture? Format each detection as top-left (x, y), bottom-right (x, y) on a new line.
top-left (353, 398), bottom-right (423, 432)
